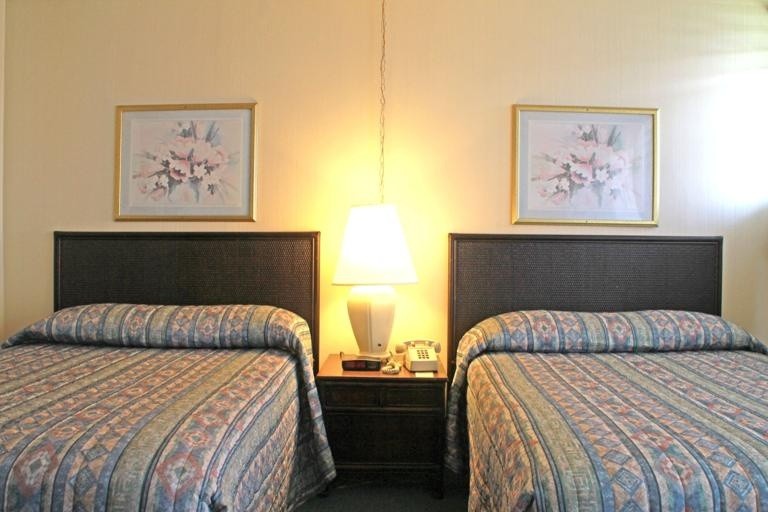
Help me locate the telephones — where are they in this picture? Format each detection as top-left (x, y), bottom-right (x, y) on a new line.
top-left (395, 339), bottom-right (441, 372)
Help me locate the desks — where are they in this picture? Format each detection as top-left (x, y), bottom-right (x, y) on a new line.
top-left (315, 352), bottom-right (448, 502)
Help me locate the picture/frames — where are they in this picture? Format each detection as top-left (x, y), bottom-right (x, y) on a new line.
top-left (511, 103), bottom-right (660, 227)
top-left (113, 103), bottom-right (257, 223)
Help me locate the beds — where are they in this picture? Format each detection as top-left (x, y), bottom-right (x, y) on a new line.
top-left (443, 309), bottom-right (767, 511)
top-left (0, 304), bottom-right (336, 512)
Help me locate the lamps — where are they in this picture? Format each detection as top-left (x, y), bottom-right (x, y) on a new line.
top-left (331, 204), bottom-right (417, 361)
